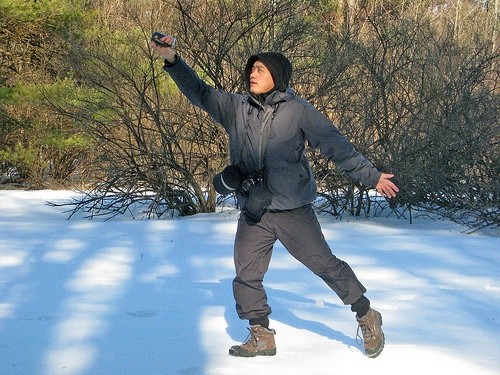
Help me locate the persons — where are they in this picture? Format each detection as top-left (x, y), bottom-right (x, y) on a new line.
top-left (150, 36), bottom-right (399, 358)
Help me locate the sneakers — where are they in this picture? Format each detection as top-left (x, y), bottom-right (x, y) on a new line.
top-left (229, 324), bottom-right (277, 357)
top-left (356, 306), bottom-right (385, 358)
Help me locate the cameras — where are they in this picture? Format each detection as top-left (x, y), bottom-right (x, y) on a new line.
top-left (239, 173), bottom-right (264, 197)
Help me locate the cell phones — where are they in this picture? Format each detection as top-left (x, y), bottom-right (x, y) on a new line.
top-left (152, 32), bottom-right (176, 47)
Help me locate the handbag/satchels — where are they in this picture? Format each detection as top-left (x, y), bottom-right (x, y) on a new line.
top-left (242, 185), bottom-right (273, 222)
